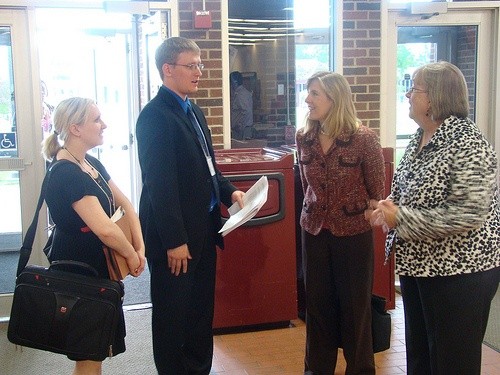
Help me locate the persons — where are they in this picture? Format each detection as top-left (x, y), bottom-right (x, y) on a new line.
top-left (363, 61), bottom-right (500, 375)
top-left (42, 97), bottom-right (146, 375)
top-left (136, 36), bottom-right (247, 375)
top-left (230, 71), bottom-right (254, 139)
top-left (296, 71), bottom-right (385, 375)
top-left (41, 80), bottom-right (55, 145)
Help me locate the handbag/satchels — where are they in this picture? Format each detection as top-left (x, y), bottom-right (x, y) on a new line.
top-left (6, 259), bottom-right (128, 361)
top-left (337, 294), bottom-right (391, 354)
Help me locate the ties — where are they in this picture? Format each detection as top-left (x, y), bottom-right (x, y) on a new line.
top-left (186, 105), bottom-right (221, 212)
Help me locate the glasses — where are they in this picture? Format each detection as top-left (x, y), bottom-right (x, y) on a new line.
top-left (408, 85), bottom-right (428, 94)
top-left (168, 62), bottom-right (205, 73)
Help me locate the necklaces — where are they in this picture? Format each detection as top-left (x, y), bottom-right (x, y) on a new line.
top-left (61, 146), bottom-right (113, 207)
top-left (321, 127), bottom-right (332, 136)
